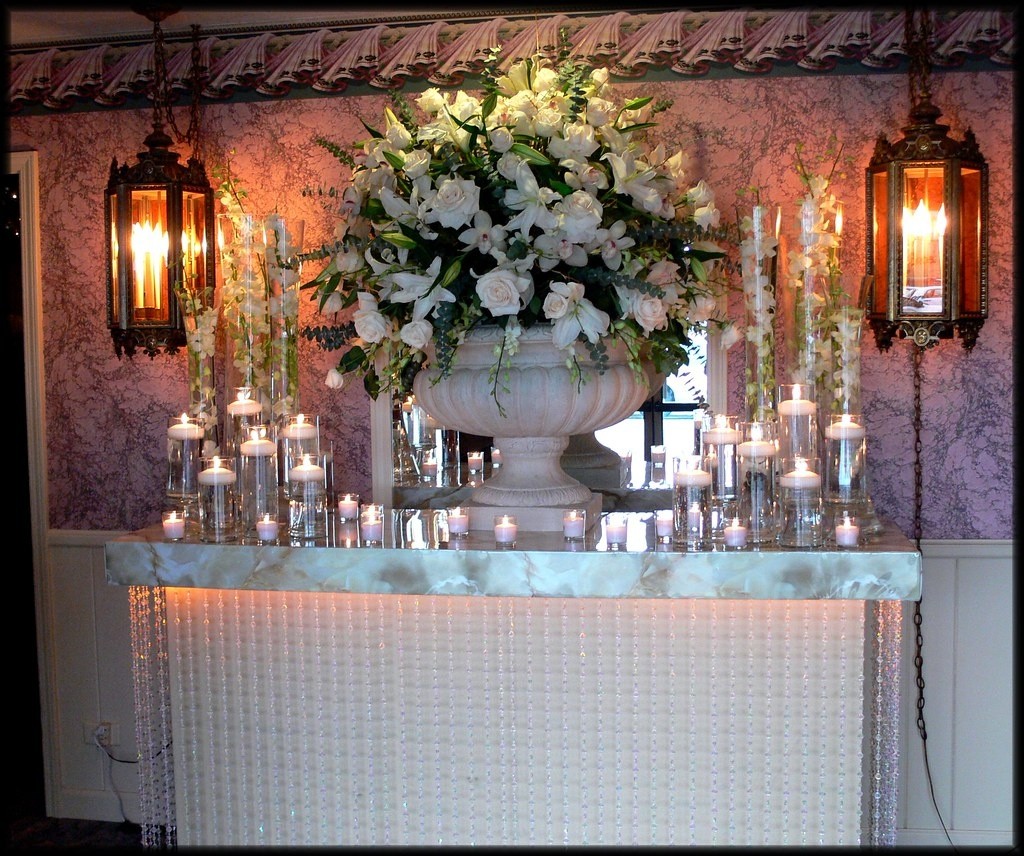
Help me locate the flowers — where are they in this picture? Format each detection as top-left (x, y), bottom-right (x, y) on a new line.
top-left (161, 250), bottom-right (218, 437)
top-left (825, 270), bottom-right (869, 412)
top-left (784, 132), bottom-right (850, 385)
top-left (211, 144), bottom-right (265, 393)
top-left (734, 187), bottom-right (774, 423)
top-left (265, 214), bottom-right (305, 404)
top-left (270, 37), bottom-right (734, 403)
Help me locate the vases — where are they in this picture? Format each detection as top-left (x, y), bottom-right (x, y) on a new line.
top-left (218, 214), bottom-right (260, 389)
top-left (791, 199), bottom-right (845, 385)
top-left (412, 331), bottom-right (652, 535)
top-left (263, 220), bottom-right (301, 414)
top-left (738, 206), bottom-right (776, 424)
top-left (819, 277), bottom-right (886, 539)
top-left (172, 289), bottom-right (218, 439)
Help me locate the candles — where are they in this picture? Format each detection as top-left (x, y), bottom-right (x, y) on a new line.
top-left (777, 384), bottom-right (817, 419)
top-left (723, 519), bottom-right (746, 547)
top-left (257, 514), bottom-right (278, 543)
top-left (282, 412), bottom-right (319, 442)
top-left (360, 506), bottom-right (382, 542)
top-left (781, 459), bottom-right (820, 486)
top-left (694, 407), bottom-right (740, 467)
top-left (563, 449), bottom-right (633, 544)
top-left (676, 461), bottom-right (712, 489)
top-left (650, 446), bottom-right (672, 536)
top-left (825, 413), bottom-right (867, 442)
top-left (836, 517), bottom-right (857, 547)
top-left (288, 453), bottom-right (328, 483)
top-left (161, 394), bottom-right (236, 541)
top-left (737, 424), bottom-right (776, 458)
top-left (490, 447), bottom-right (517, 545)
top-left (338, 493), bottom-right (359, 521)
top-left (228, 389), bottom-right (262, 415)
top-left (242, 429), bottom-right (276, 456)
top-left (395, 400), bottom-right (484, 538)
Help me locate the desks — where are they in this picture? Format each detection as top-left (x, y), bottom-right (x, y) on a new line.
top-left (105, 525), bottom-right (924, 848)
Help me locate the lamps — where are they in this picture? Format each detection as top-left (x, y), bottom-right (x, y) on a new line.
top-left (100, 125), bottom-right (214, 362)
top-left (860, 105), bottom-right (990, 354)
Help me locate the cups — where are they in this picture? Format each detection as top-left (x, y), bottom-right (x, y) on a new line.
top-left (320, 440), bottom-right (335, 513)
